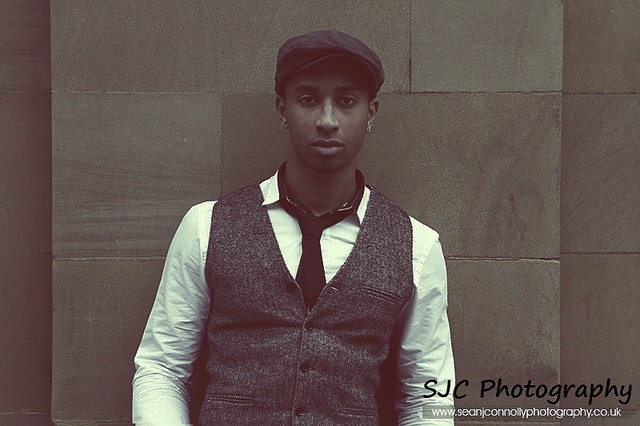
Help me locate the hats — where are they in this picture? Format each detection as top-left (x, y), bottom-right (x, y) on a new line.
top-left (274, 30), bottom-right (386, 97)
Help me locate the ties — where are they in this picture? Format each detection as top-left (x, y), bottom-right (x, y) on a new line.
top-left (274, 154), bottom-right (365, 312)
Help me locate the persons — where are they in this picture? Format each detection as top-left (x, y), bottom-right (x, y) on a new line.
top-left (130, 29), bottom-right (458, 425)
